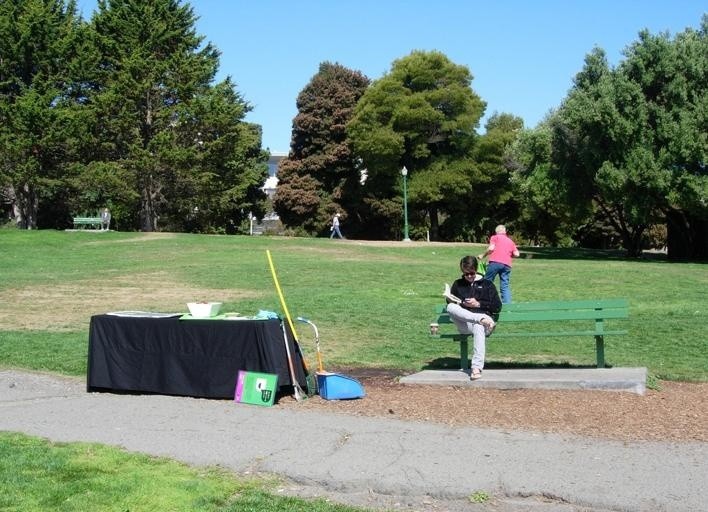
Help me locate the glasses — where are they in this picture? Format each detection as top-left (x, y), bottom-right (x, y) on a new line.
top-left (463, 272), bottom-right (475, 276)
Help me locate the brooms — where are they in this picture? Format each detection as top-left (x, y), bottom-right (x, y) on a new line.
top-left (281, 320), bottom-right (308, 402)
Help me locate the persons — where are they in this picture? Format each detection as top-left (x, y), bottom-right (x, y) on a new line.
top-left (476, 223), bottom-right (520, 303)
top-left (445, 255), bottom-right (502, 379)
top-left (329, 213), bottom-right (344, 240)
top-left (100, 205), bottom-right (112, 232)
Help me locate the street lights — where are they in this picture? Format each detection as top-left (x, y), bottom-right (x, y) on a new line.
top-left (401, 166), bottom-right (411, 241)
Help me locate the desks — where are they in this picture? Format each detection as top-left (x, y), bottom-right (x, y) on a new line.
top-left (88, 312), bottom-right (301, 406)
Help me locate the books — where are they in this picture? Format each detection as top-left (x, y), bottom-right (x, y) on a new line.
top-left (442, 283), bottom-right (461, 305)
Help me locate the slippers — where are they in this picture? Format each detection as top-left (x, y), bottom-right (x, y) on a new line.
top-left (487, 321), bottom-right (498, 331)
top-left (470, 371), bottom-right (481, 380)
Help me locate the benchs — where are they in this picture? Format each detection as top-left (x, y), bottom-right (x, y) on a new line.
top-left (73, 217), bottom-right (104, 231)
top-left (433, 297), bottom-right (629, 369)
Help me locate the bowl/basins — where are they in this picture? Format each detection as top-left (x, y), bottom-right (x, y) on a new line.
top-left (186, 302), bottom-right (223, 318)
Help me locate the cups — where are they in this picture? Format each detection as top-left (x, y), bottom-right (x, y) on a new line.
top-left (430, 324), bottom-right (439, 335)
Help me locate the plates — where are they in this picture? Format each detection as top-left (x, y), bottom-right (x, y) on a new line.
top-left (224, 313), bottom-right (240, 317)
